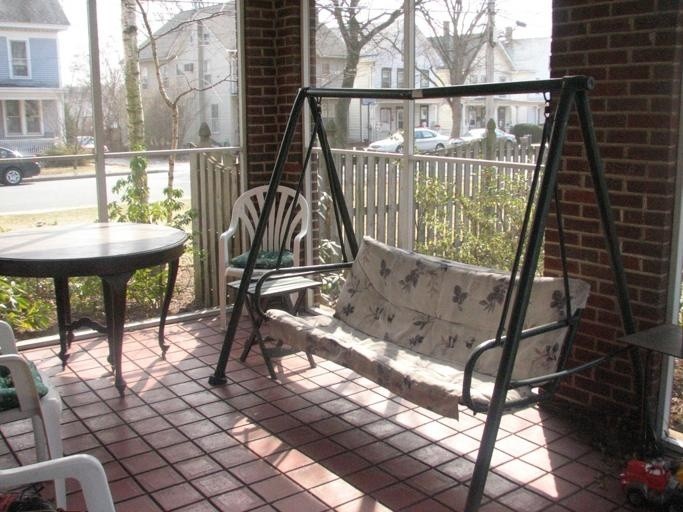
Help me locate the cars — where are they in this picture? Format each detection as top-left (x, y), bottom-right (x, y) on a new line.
top-left (0, 147), bottom-right (40, 184)
top-left (461, 128), bottom-right (517, 149)
top-left (368, 126), bottom-right (449, 155)
top-left (49, 134), bottom-right (110, 154)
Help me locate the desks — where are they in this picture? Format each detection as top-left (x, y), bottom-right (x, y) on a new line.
top-left (0, 222), bottom-right (189, 397)
top-left (615, 324), bottom-right (683, 460)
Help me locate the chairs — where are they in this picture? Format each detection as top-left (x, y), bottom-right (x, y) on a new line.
top-left (219, 184), bottom-right (309, 331)
top-left (0, 453), bottom-right (116, 512)
top-left (0, 320), bottom-right (62, 458)
